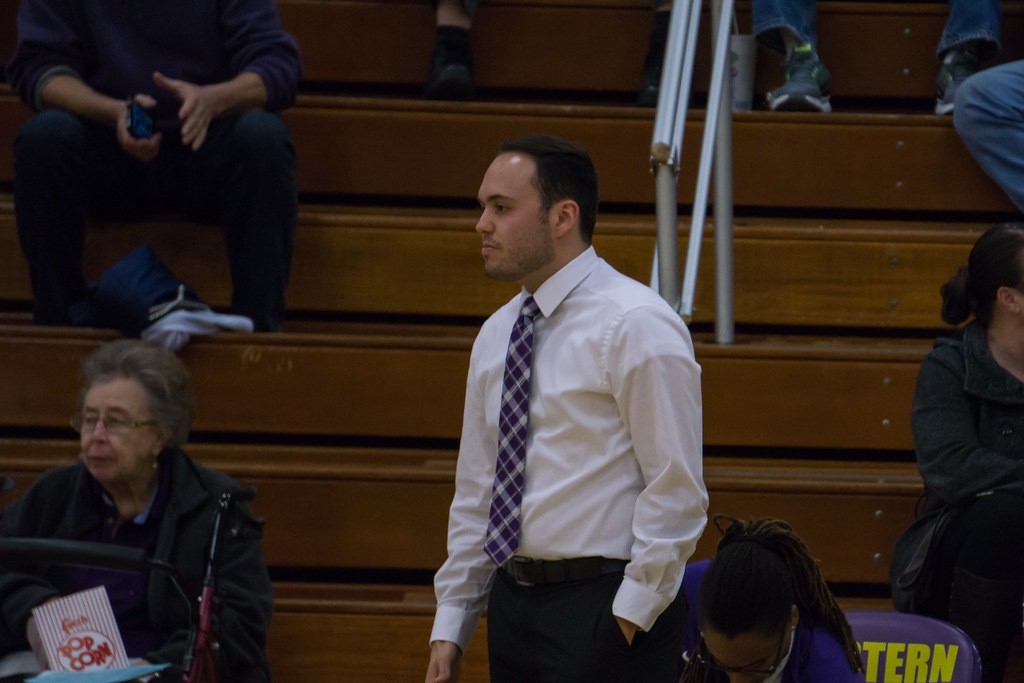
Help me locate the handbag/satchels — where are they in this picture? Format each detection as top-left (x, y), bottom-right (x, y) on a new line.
top-left (891, 494), bottom-right (970, 622)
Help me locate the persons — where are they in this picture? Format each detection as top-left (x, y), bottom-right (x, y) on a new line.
top-left (954, 58), bottom-right (1024, 212)
top-left (428, 0), bottom-right (695, 105)
top-left (424, 133), bottom-right (710, 683)
top-left (751, 0), bottom-right (1002, 114)
top-left (909, 223), bottom-right (1024, 683)
top-left (0, 339), bottom-right (274, 683)
top-left (7, 0), bottom-right (303, 332)
top-left (678, 513), bottom-right (866, 683)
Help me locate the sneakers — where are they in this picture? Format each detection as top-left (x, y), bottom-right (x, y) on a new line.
top-left (934, 50), bottom-right (985, 115)
top-left (766, 41), bottom-right (833, 113)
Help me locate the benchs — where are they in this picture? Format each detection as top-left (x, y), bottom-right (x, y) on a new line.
top-left (0, 0), bottom-right (1022, 683)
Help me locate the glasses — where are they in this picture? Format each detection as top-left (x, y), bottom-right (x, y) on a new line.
top-left (696, 622), bottom-right (786, 674)
top-left (68, 411), bottom-right (158, 433)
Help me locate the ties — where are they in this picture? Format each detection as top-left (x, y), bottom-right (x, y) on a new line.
top-left (483, 295), bottom-right (543, 568)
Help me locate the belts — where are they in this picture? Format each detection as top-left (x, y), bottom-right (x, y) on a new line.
top-left (503, 555), bottom-right (631, 587)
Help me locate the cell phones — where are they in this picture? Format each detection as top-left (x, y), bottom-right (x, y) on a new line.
top-left (126, 97), bottom-right (156, 141)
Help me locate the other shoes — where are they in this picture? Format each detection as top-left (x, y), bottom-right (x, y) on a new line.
top-left (424, 55), bottom-right (477, 102)
top-left (639, 68), bottom-right (697, 108)
